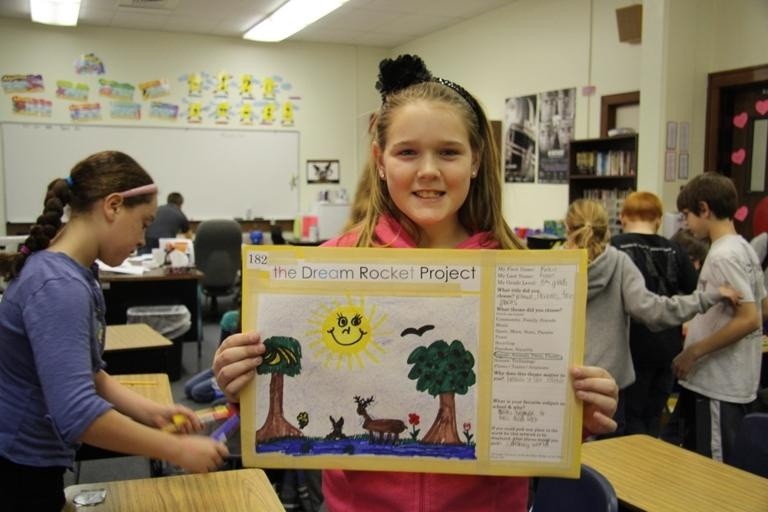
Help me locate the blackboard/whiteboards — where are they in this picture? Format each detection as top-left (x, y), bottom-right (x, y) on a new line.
top-left (0, 120), bottom-right (300, 224)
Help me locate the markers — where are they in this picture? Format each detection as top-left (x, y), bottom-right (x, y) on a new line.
top-left (159, 406), bottom-right (240, 444)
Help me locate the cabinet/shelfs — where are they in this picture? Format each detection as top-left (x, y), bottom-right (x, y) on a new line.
top-left (567, 134), bottom-right (638, 239)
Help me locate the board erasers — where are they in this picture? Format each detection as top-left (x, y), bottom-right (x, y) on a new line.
top-left (254, 218), bottom-right (263, 221)
top-left (233, 218), bottom-right (243, 221)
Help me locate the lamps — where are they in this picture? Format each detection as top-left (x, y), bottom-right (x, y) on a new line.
top-left (30, 0), bottom-right (81, 27)
top-left (242, 0), bottom-right (349, 43)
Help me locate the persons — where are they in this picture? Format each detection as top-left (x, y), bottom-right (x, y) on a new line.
top-left (559, 171), bottom-right (768, 472)
top-left (212, 52), bottom-right (618, 512)
top-left (0, 151), bottom-right (231, 512)
top-left (184, 307), bottom-right (241, 403)
top-left (138, 192), bottom-right (189, 256)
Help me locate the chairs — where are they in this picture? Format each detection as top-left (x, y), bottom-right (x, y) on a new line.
top-left (194, 219), bottom-right (242, 323)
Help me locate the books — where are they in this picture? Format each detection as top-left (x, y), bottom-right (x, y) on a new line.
top-left (575, 150), bottom-right (636, 217)
